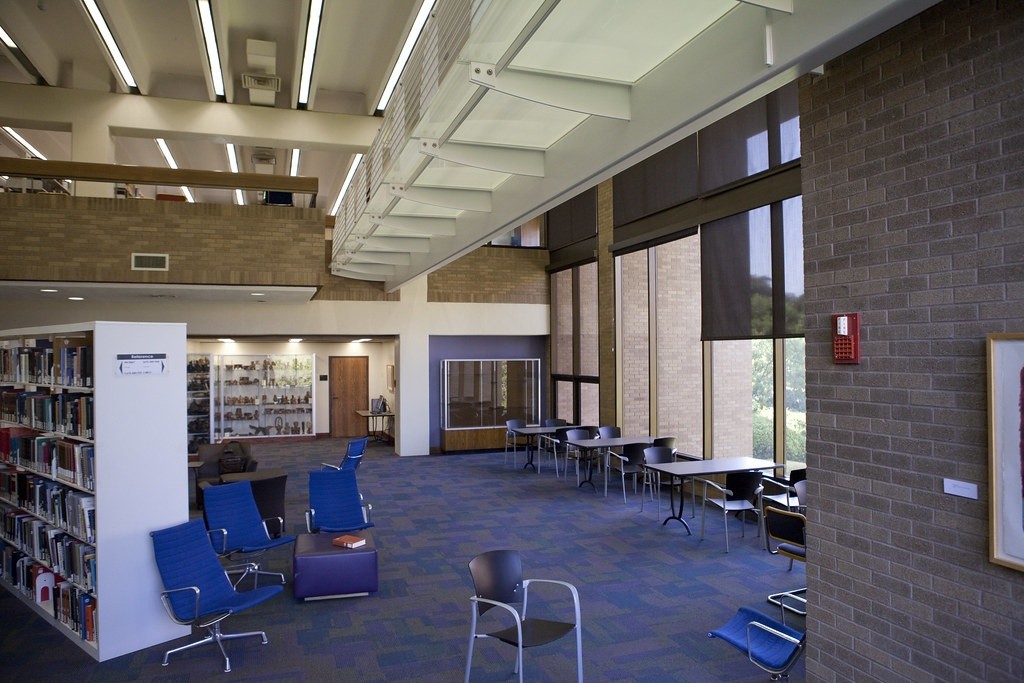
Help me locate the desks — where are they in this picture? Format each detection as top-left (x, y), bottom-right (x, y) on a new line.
top-left (642, 456), bottom-right (785, 533)
top-left (512, 425), bottom-right (574, 472)
top-left (357, 410), bottom-right (393, 444)
top-left (563, 435), bottom-right (659, 494)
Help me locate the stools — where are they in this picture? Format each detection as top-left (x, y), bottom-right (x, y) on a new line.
top-left (296, 533), bottom-right (378, 603)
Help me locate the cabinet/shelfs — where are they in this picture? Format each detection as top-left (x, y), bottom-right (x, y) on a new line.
top-left (0, 321), bottom-right (191, 651)
top-left (187, 352), bottom-right (317, 444)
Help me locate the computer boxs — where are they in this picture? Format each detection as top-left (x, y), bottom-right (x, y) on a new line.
top-left (371, 399), bottom-right (387, 413)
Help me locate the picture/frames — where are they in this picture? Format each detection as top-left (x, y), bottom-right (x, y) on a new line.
top-left (985, 333), bottom-right (1024, 574)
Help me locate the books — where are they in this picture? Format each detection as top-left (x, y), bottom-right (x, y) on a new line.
top-left (0, 540), bottom-right (96, 641)
top-left (0, 345), bottom-right (93, 390)
top-left (333, 535), bottom-right (366, 548)
top-left (0, 502), bottom-right (96, 595)
top-left (0, 426), bottom-right (94, 492)
top-left (0, 386), bottom-right (94, 442)
top-left (0, 464), bottom-right (95, 544)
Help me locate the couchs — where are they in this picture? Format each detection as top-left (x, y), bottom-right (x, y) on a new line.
top-left (201, 468), bottom-right (291, 536)
top-left (189, 442), bottom-right (255, 512)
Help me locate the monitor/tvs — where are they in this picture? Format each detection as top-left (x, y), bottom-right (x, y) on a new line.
top-left (374, 395), bottom-right (384, 414)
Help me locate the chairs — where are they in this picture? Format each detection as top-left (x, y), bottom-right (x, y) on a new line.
top-left (463, 549), bottom-right (587, 683)
top-left (503, 418), bottom-right (807, 566)
top-left (146, 436), bottom-right (374, 675)
top-left (709, 593), bottom-right (806, 683)
